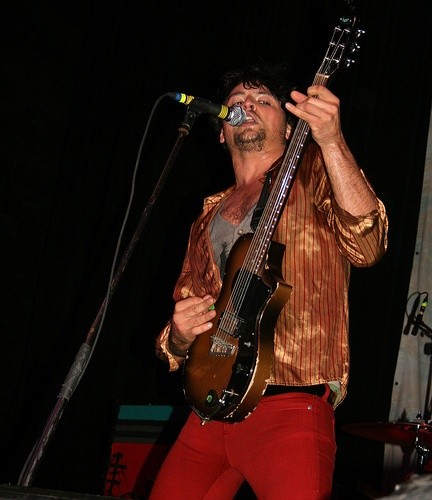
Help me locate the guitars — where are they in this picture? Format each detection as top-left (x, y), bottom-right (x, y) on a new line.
top-left (180, 10), bottom-right (367, 425)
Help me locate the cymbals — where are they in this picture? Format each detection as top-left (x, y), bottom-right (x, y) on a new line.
top-left (353, 419), bottom-right (432, 447)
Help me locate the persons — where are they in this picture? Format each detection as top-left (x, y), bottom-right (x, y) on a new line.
top-left (133, 64), bottom-right (393, 499)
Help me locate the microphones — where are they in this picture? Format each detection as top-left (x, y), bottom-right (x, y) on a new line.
top-left (412, 296), bottom-right (428, 336)
top-left (167, 92), bottom-right (247, 127)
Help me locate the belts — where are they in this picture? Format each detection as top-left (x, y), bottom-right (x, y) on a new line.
top-left (263, 383), bottom-right (335, 404)
